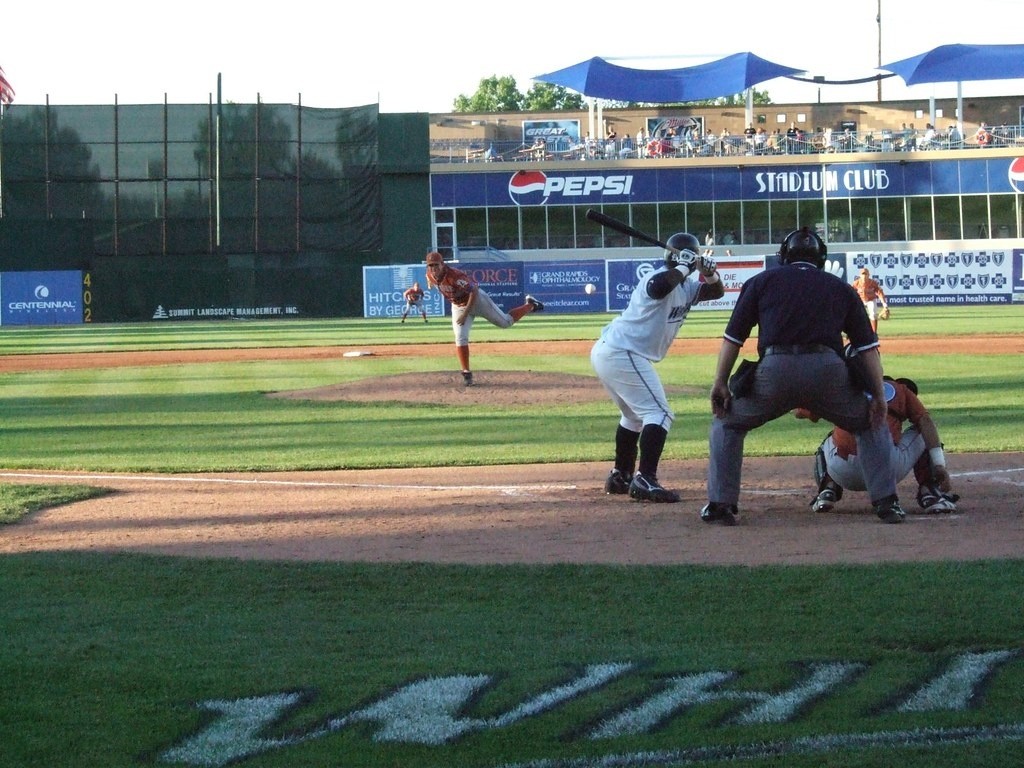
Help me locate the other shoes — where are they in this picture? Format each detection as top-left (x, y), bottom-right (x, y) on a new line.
top-left (701, 501), bottom-right (743, 527)
top-left (603, 469), bottom-right (634, 495)
top-left (401, 320), bottom-right (405, 323)
top-left (877, 498), bottom-right (913, 523)
top-left (627, 473), bottom-right (678, 501)
top-left (425, 320), bottom-right (428, 323)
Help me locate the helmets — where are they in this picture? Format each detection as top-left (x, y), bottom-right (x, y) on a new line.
top-left (777, 227), bottom-right (835, 268)
top-left (662, 233), bottom-right (702, 265)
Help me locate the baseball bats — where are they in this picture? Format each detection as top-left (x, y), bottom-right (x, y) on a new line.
top-left (584, 207), bottom-right (681, 256)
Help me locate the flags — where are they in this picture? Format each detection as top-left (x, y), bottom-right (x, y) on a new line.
top-left (0, 66), bottom-right (16, 111)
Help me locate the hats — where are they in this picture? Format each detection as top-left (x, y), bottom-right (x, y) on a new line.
top-left (859, 268), bottom-right (870, 277)
top-left (426, 252), bottom-right (444, 265)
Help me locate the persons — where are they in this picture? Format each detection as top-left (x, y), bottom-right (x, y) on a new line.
top-left (645, 130), bottom-right (654, 147)
top-left (794, 374), bottom-right (960, 512)
top-left (532, 138), bottom-right (547, 152)
top-left (666, 126), bottom-right (718, 157)
top-left (567, 137), bottom-right (575, 147)
top-left (400, 282), bottom-right (428, 323)
top-left (705, 228), bottom-right (714, 246)
top-left (864, 122), bottom-right (962, 151)
top-left (816, 126), bottom-right (853, 153)
top-left (851, 268), bottom-right (890, 334)
top-left (636, 127), bottom-right (645, 159)
top-left (721, 128), bottom-right (733, 156)
top-left (426, 251), bottom-right (544, 386)
top-left (744, 122), bottom-right (807, 155)
top-left (590, 232), bottom-right (727, 503)
top-left (700, 225), bottom-right (910, 524)
top-left (604, 126), bottom-right (633, 159)
top-left (585, 132), bottom-right (589, 143)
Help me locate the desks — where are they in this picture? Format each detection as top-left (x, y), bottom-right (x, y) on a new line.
top-left (468, 150), bottom-right (485, 153)
top-left (519, 149), bottom-right (540, 162)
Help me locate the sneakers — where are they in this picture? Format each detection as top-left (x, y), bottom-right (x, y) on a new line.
top-left (526, 295), bottom-right (544, 310)
top-left (919, 483), bottom-right (959, 514)
top-left (807, 488), bottom-right (839, 514)
top-left (461, 372), bottom-right (474, 387)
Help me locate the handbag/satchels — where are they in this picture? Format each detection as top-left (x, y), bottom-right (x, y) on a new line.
top-left (730, 358), bottom-right (759, 398)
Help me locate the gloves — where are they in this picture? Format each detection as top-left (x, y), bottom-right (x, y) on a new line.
top-left (700, 254), bottom-right (719, 275)
top-left (676, 249), bottom-right (699, 271)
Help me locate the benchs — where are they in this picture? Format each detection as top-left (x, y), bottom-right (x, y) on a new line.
top-left (467, 156), bottom-right (504, 162)
top-left (512, 154), bottom-right (553, 162)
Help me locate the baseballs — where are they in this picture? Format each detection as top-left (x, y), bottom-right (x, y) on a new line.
top-left (584, 283), bottom-right (596, 294)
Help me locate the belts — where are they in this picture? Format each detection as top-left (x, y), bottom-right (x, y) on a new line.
top-left (758, 343), bottom-right (835, 356)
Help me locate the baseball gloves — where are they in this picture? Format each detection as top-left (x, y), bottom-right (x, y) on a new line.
top-left (880, 307), bottom-right (890, 320)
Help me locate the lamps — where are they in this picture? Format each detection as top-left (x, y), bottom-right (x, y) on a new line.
top-left (518, 170), bottom-right (526, 176)
top-left (737, 165), bottom-right (744, 169)
top-left (900, 160), bottom-right (905, 165)
top-left (436, 122), bottom-right (442, 126)
